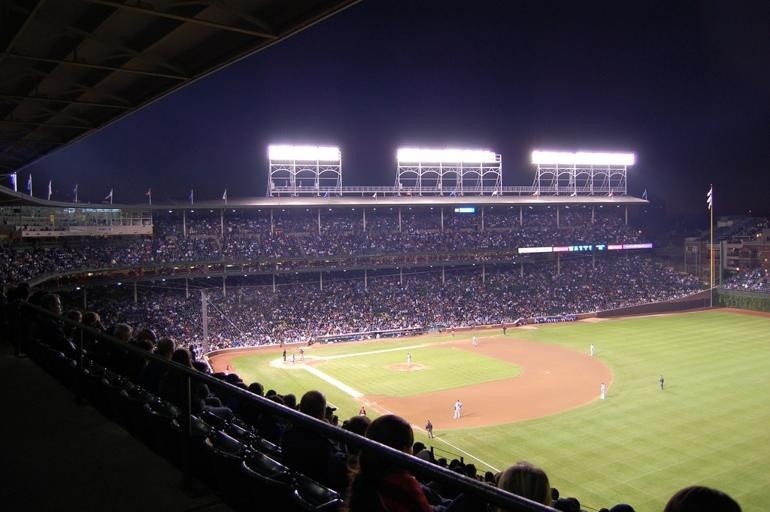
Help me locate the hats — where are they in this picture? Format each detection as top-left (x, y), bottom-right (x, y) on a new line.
top-left (228, 374), bottom-right (243, 382)
top-left (327, 406), bottom-right (337, 411)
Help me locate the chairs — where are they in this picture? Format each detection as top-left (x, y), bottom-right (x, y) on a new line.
top-left (44, 334), bottom-right (343, 510)
